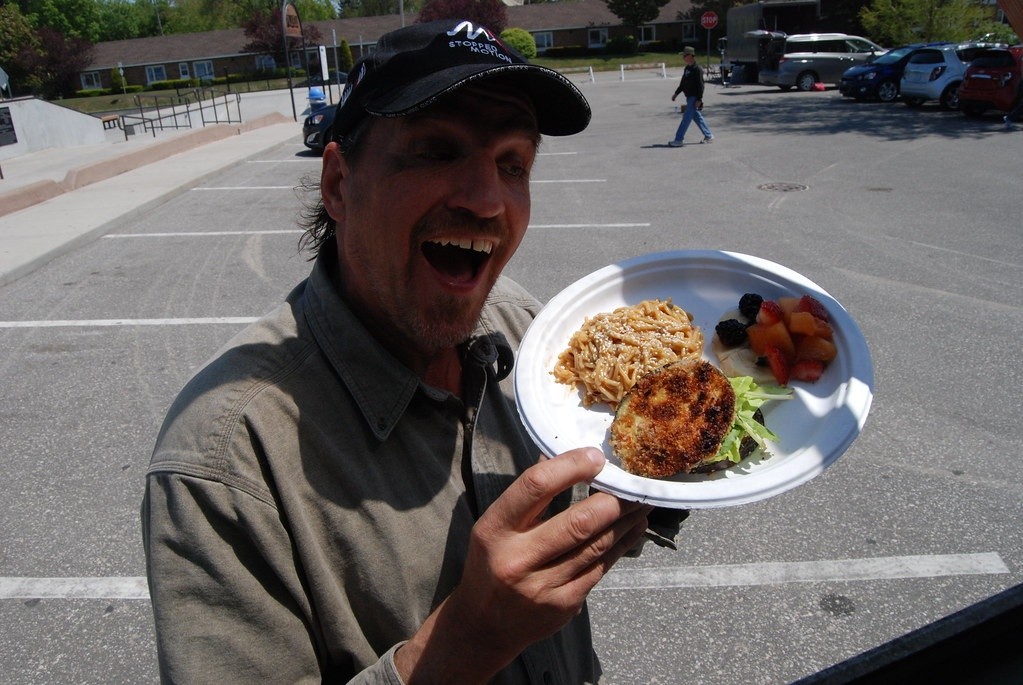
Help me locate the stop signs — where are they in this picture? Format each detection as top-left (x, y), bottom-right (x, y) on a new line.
top-left (701, 12), bottom-right (718, 29)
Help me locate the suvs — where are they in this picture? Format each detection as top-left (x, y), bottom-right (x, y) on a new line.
top-left (757, 34), bottom-right (893, 92)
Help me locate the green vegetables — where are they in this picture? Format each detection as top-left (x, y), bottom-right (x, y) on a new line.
top-left (692, 374), bottom-right (795, 463)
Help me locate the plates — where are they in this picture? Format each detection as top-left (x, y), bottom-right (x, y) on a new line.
top-left (513, 249), bottom-right (875, 511)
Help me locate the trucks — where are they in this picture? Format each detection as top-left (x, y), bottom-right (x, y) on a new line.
top-left (719, 0), bottom-right (821, 85)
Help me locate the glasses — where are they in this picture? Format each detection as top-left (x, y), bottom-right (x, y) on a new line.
top-left (684, 53), bottom-right (690, 57)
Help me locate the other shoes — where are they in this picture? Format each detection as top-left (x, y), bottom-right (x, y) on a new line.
top-left (669, 140), bottom-right (684, 147)
top-left (700, 135), bottom-right (713, 143)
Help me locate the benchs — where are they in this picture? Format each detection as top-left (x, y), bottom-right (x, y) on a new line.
top-left (102, 115), bottom-right (119, 130)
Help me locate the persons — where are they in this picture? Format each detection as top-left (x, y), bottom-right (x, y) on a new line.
top-left (668, 45), bottom-right (715, 146)
top-left (141, 18), bottom-right (650, 684)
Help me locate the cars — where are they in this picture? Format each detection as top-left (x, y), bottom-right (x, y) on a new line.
top-left (838, 42), bottom-right (938, 104)
top-left (956, 46), bottom-right (1023, 123)
top-left (302, 103), bottom-right (340, 155)
top-left (898, 42), bottom-right (1012, 111)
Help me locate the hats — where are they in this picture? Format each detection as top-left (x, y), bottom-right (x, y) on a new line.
top-left (679, 47), bottom-right (695, 55)
top-left (334, 18), bottom-right (591, 136)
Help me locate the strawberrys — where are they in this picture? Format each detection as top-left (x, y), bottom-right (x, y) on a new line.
top-left (758, 295), bottom-right (830, 386)
